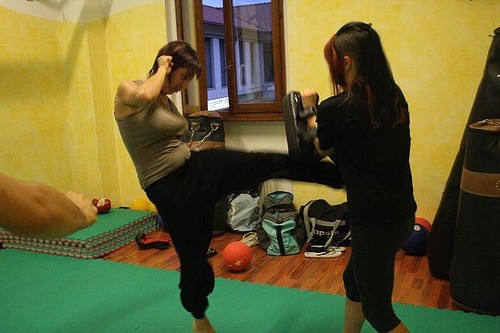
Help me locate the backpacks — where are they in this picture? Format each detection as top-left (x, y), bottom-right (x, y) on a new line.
top-left (261, 190), bottom-right (300, 255)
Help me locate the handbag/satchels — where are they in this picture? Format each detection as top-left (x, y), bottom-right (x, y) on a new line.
top-left (300, 198), bottom-right (352, 252)
top-left (226, 192), bottom-right (260, 232)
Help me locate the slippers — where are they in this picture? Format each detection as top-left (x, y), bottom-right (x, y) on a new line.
top-left (239, 232), bottom-right (259, 243)
top-left (312, 245), bottom-right (346, 251)
top-left (304, 251), bottom-right (342, 257)
top-left (246, 236), bottom-right (262, 247)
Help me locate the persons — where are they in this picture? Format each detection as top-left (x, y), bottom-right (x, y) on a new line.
top-left (2, 172), bottom-right (100, 239)
top-left (282, 20), bottom-right (419, 333)
top-left (113, 41), bottom-right (343, 332)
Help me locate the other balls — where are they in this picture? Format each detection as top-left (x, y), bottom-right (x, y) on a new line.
top-left (402, 217), bottom-right (432, 255)
top-left (224, 242), bottom-right (251, 270)
